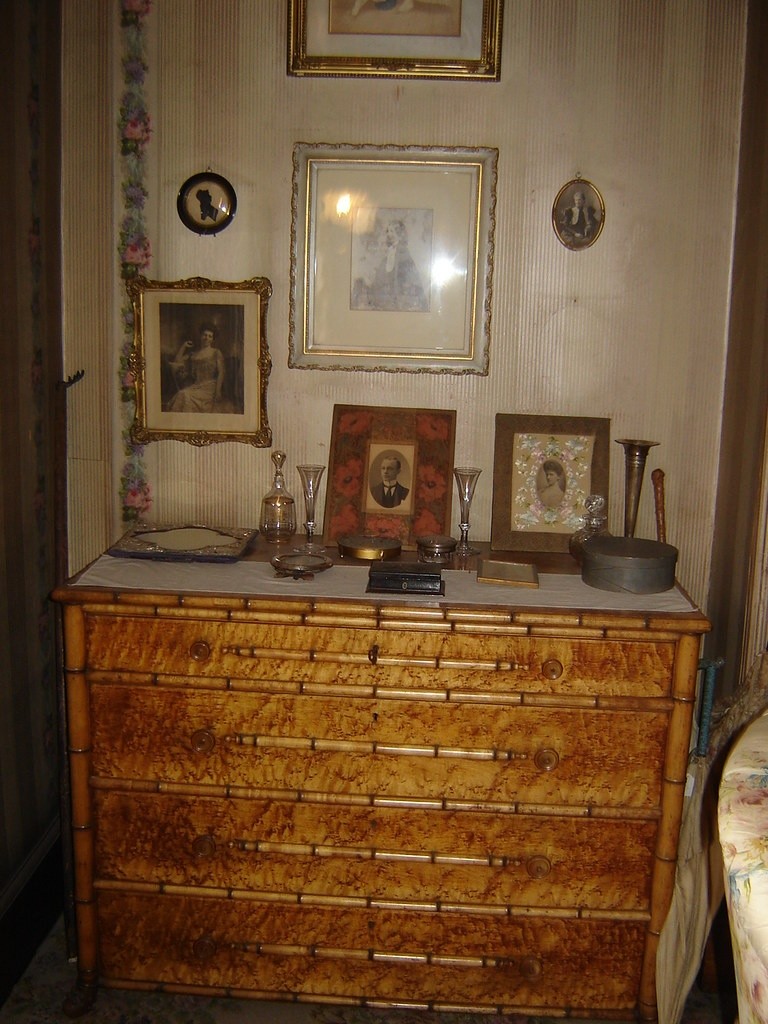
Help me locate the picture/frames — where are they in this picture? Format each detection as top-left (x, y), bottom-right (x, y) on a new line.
top-left (285, 0), bottom-right (505, 82)
top-left (288, 140), bottom-right (500, 376)
top-left (176, 172), bottom-right (238, 235)
top-left (490, 413), bottom-right (612, 555)
top-left (552, 179), bottom-right (605, 252)
top-left (476, 557), bottom-right (539, 589)
top-left (125, 274), bottom-right (273, 450)
top-left (323, 405), bottom-right (457, 552)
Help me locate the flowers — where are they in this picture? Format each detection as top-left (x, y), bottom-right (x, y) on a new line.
top-left (117, 0), bottom-right (155, 521)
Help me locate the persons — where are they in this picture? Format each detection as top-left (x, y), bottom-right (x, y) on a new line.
top-left (166, 329), bottom-right (224, 411)
top-left (372, 219), bottom-right (427, 310)
top-left (371, 457), bottom-right (410, 508)
top-left (561, 193), bottom-right (595, 246)
top-left (538, 460), bottom-right (564, 506)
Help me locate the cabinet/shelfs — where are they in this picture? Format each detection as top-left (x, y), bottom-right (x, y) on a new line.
top-left (47, 522), bottom-right (712, 1023)
